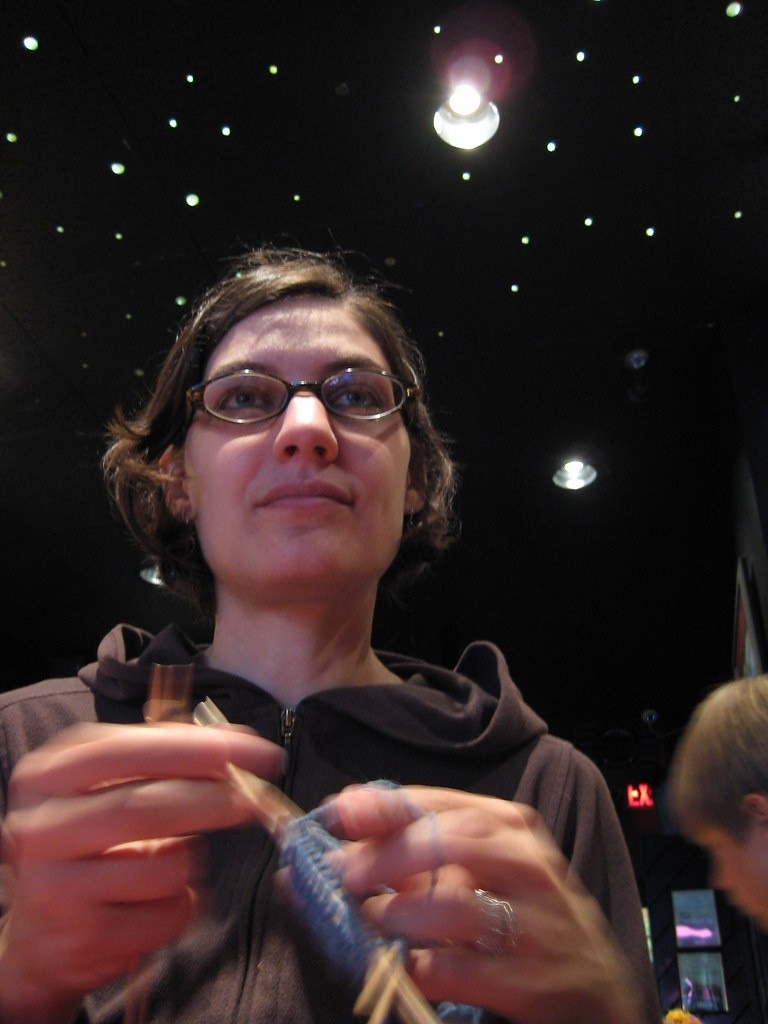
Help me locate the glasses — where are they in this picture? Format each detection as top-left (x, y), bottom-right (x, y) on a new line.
top-left (181, 366), bottom-right (419, 440)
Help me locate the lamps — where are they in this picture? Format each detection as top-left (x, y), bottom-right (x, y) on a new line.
top-left (429, 83), bottom-right (502, 152)
top-left (138, 552), bottom-right (164, 585)
top-left (552, 443), bottom-right (597, 492)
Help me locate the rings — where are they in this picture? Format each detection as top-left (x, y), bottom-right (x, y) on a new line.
top-left (474, 891), bottom-right (520, 959)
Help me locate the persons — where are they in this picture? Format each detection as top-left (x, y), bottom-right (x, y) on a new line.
top-left (0, 247), bottom-right (657, 1024)
top-left (670, 676), bottom-right (768, 937)
